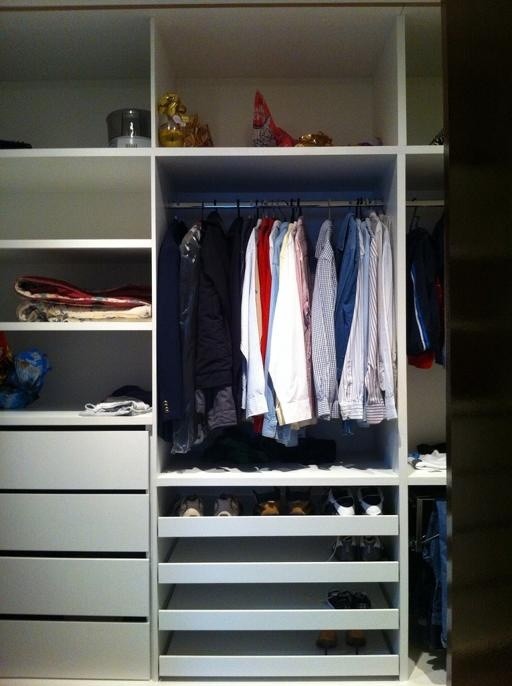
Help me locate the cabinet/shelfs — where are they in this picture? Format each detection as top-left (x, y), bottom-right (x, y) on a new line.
top-left (403, 6), bottom-right (447, 680)
top-left (0, 6), bottom-right (154, 426)
top-left (0, 427), bottom-right (150, 587)
top-left (155, 1), bottom-right (402, 475)
top-left (150, 472), bottom-right (407, 676)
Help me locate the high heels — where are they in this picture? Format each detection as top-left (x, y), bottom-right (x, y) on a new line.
top-left (316, 630), bottom-right (365, 655)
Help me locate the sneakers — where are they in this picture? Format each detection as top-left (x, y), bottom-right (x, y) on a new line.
top-left (332, 537), bottom-right (384, 561)
top-left (328, 590), bottom-right (370, 609)
top-left (170, 486), bottom-right (384, 517)
